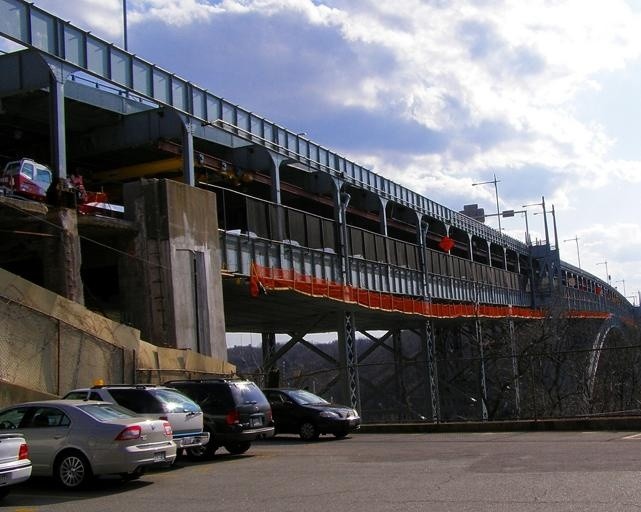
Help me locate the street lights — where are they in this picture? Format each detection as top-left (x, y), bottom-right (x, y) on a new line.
top-left (522, 196), bottom-right (559, 249)
top-left (563, 234), bottom-right (580, 269)
top-left (615, 280), bottom-right (626, 296)
top-left (472, 173), bottom-right (502, 233)
top-left (596, 260), bottom-right (609, 284)
top-left (630, 290), bottom-right (641, 306)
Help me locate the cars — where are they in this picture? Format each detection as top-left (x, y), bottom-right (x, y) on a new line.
top-left (0, 380), bottom-right (211, 491)
top-left (261, 388), bottom-right (358, 440)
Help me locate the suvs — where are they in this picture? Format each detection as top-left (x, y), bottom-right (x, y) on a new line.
top-left (161, 381), bottom-right (276, 461)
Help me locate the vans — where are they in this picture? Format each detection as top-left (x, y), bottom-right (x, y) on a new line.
top-left (2, 157), bottom-right (53, 199)
